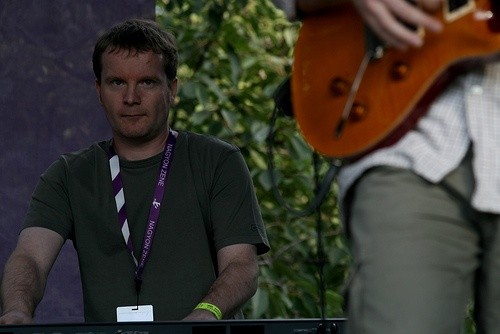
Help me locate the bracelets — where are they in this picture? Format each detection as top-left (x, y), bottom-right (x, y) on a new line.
top-left (193, 303), bottom-right (222, 320)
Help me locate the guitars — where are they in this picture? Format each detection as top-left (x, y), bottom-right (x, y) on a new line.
top-left (291, 0), bottom-right (500, 165)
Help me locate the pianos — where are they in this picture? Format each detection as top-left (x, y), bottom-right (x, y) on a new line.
top-left (0, 318), bottom-right (348, 334)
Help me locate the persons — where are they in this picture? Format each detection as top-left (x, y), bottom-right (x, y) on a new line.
top-left (271, 0), bottom-right (500, 334)
top-left (0, 19), bottom-right (271, 326)
